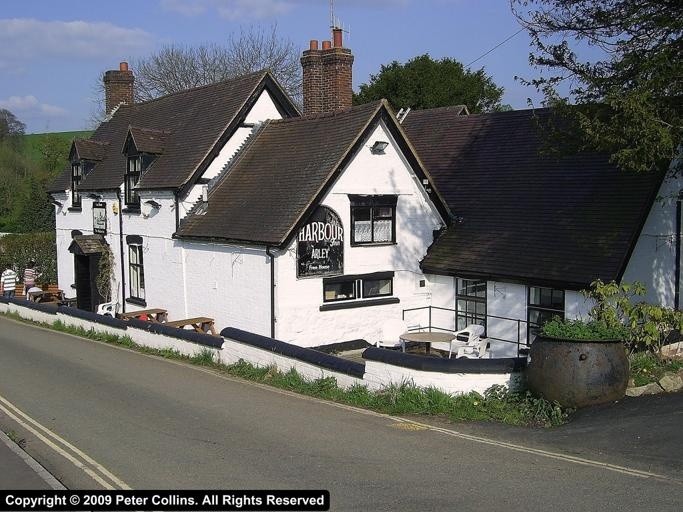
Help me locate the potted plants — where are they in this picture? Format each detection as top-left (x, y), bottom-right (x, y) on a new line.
top-left (524, 312), bottom-right (628, 409)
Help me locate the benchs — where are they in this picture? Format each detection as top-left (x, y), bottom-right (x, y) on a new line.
top-left (27, 290), bottom-right (216, 336)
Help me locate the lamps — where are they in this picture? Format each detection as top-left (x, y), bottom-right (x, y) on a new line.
top-left (51, 201), bottom-right (62, 207)
top-left (87, 193), bottom-right (104, 201)
top-left (145, 200), bottom-right (161, 209)
top-left (370, 141), bottom-right (389, 152)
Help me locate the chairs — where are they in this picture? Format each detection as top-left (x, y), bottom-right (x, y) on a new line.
top-left (456, 338), bottom-right (490, 359)
top-left (377, 319), bottom-right (407, 353)
top-left (447, 324), bottom-right (484, 359)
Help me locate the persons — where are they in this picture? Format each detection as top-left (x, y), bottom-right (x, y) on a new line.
top-left (0, 263), bottom-right (20, 299)
top-left (22, 260), bottom-right (43, 297)
top-left (25, 284), bottom-right (44, 304)
